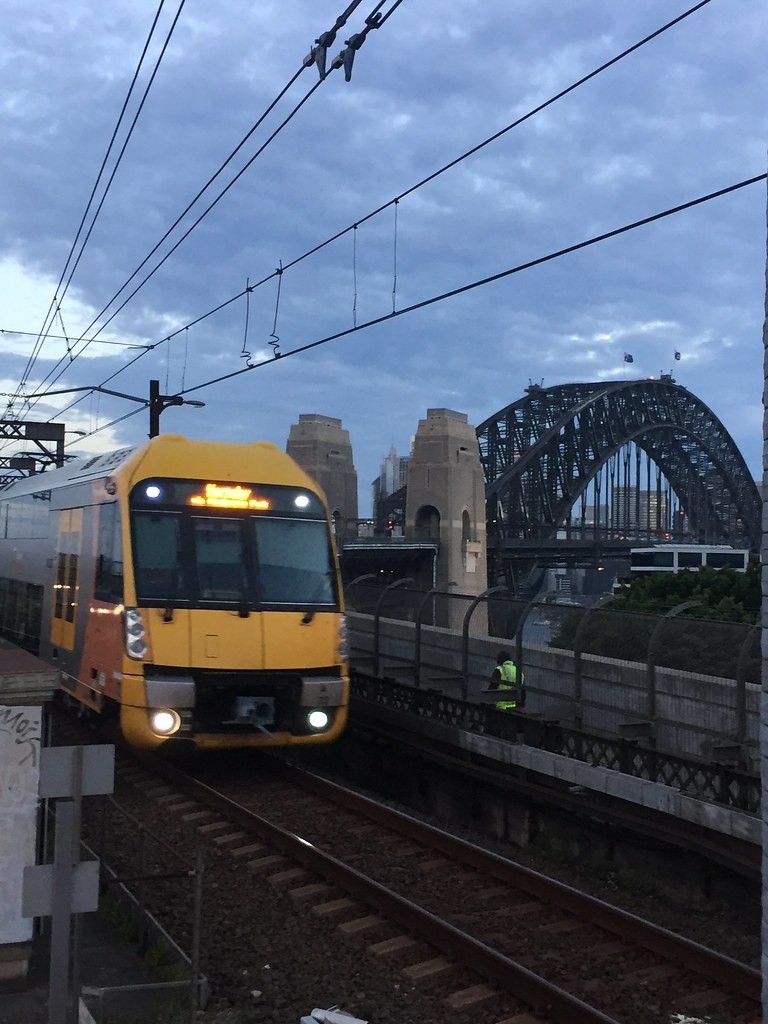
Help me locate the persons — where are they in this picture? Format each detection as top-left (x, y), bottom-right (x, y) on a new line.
top-left (485, 650), bottom-right (526, 741)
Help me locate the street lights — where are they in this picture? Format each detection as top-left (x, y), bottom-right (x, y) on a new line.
top-left (150, 377), bottom-right (206, 435)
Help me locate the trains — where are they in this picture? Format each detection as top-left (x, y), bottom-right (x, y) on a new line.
top-left (0, 433), bottom-right (351, 773)
